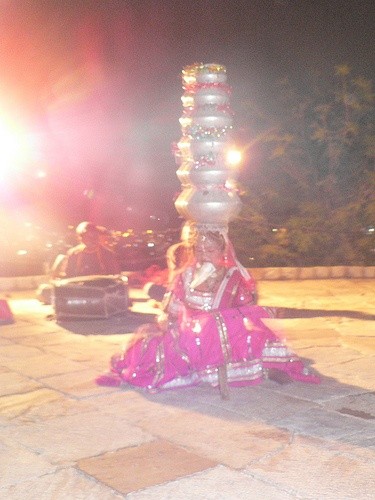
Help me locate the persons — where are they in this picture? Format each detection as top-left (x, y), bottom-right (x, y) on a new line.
top-left (53, 222), bottom-right (119, 280)
top-left (99, 231), bottom-right (319, 391)
